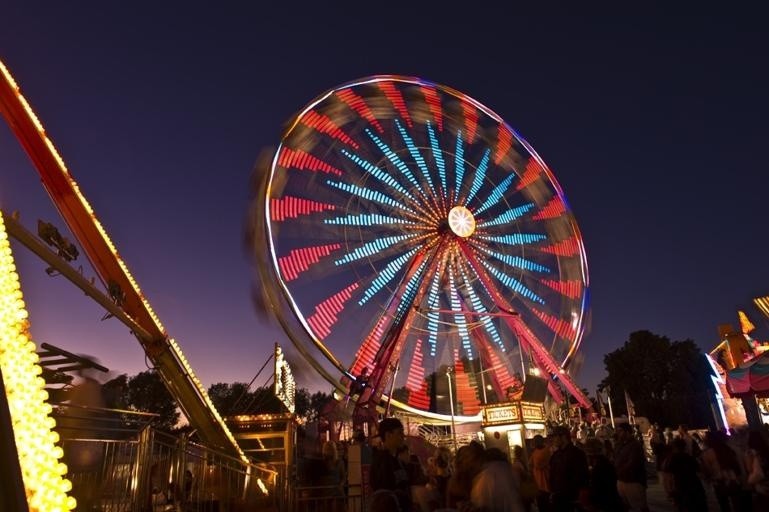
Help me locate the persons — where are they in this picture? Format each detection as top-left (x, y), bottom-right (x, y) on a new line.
top-left (369, 419), bottom-right (769, 511)
top-left (314, 441), bottom-right (347, 511)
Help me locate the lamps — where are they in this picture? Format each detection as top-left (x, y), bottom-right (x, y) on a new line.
top-left (34, 220), bottom-right (127, 324)
